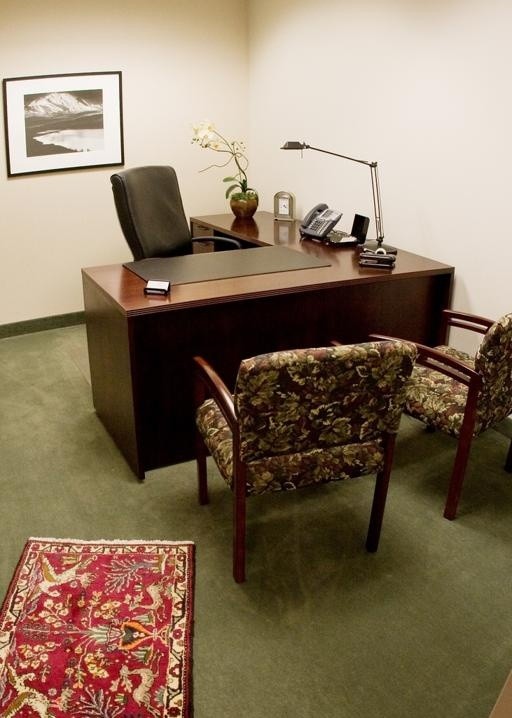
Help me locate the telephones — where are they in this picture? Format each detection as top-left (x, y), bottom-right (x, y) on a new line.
top-left (299, 203), bottom-right (343, 237)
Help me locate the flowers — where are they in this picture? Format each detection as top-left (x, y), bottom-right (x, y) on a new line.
top-left (189, 117), bottom-right (259, 201)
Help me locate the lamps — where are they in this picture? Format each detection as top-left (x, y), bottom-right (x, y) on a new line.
top-left (280, 140), bottom-right (398, 256)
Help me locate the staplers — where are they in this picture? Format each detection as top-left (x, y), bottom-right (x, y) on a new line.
top-left (358, 252), bottom-right (396, 269)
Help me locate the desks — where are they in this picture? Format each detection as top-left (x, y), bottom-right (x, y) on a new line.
top-left (81, 211), bottom-right (455, 481)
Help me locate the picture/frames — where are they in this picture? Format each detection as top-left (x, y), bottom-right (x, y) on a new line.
top-left (3, 71), bottom-right (124, 176)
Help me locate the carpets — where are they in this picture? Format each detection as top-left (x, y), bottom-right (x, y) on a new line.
top-left (0, 535), bottom-right (196, 718)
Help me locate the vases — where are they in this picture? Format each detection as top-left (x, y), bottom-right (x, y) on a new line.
top-left (228, 193), bottom-right (259, 218)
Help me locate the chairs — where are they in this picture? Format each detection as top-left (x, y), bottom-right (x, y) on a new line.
top-left (369, 308), bottom-right (512, 522)
top-left (190, 340), bottom-right (417, 585)
top-left (110, 164), bottom-right (241, 264)
